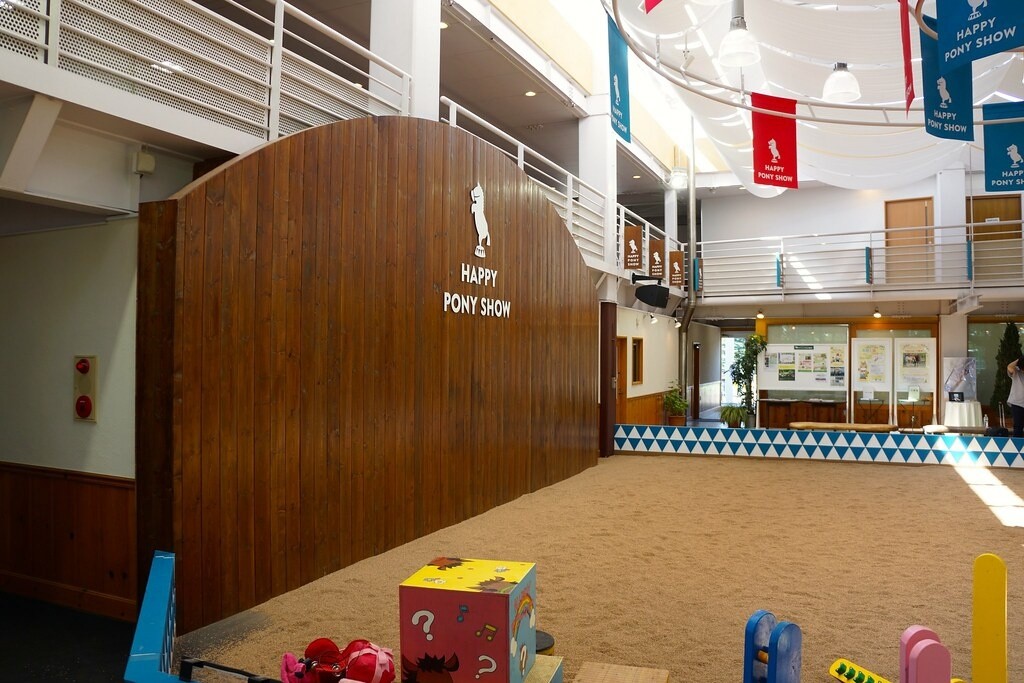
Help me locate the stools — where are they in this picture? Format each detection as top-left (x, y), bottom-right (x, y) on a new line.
top-left (923, 424), bottom-right (948, 435)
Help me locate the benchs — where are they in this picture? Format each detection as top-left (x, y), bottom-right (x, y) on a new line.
top-left (789, 421), bottom-right (899, 433)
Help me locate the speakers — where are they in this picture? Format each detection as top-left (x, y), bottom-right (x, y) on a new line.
top-left (635, 285), bottom-right (669, 309)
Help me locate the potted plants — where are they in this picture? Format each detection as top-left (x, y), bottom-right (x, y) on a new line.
top-left (730, 333), bottom-right (767, 429)
top-left (720, 406), bottom-right (749, 428)
top-left (664, 379), bottom-right (689, 426)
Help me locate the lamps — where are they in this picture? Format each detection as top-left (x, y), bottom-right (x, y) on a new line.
top-left (717, 0), bottom-right (761, 68)
top-left (756, 310), bottom-right (765, 320)
top-left (649, 314), bottom-right (658, 325)
top-left (908, 385), bottom-right (920, 434)
top-left (669, 146), bottom-right (688, 190)
top-left (822, 62), bottom-right (861, 104)
top-left (631, 271), bottom-right (670, 309)
top-left (673, 318), bottom-right (682, 328)
top-left (680, 35), bottom-right (695, 71)
top-left (873, 307), bottom-right (881, 319)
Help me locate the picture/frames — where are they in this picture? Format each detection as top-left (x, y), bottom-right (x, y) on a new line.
top-left (949, 392), bottom-right (965, 403)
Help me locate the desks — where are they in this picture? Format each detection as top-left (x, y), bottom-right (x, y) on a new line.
top-left (899, 428), bottom-right (924, 434)
top-left (944, 400), bottom-right (984, 437)
top-left (759, 398), bottom-right (930, 435)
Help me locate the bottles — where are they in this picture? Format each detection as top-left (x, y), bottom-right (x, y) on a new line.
top-left (984, 414), bottom-right (989, 427)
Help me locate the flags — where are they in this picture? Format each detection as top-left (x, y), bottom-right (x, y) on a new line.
top-left (751, 91), bottom-right (797, 190)
top-left (607, 12), bottom-right (630, 145)
top-left (900, 0), bottom-right (1024, 193)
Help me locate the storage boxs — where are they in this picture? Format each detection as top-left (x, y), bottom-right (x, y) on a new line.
top-left (398, 556), bottom-right (565, 683)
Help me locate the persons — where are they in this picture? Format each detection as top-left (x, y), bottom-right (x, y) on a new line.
top-left (1006, 357), bottom-right (1024, 437)
